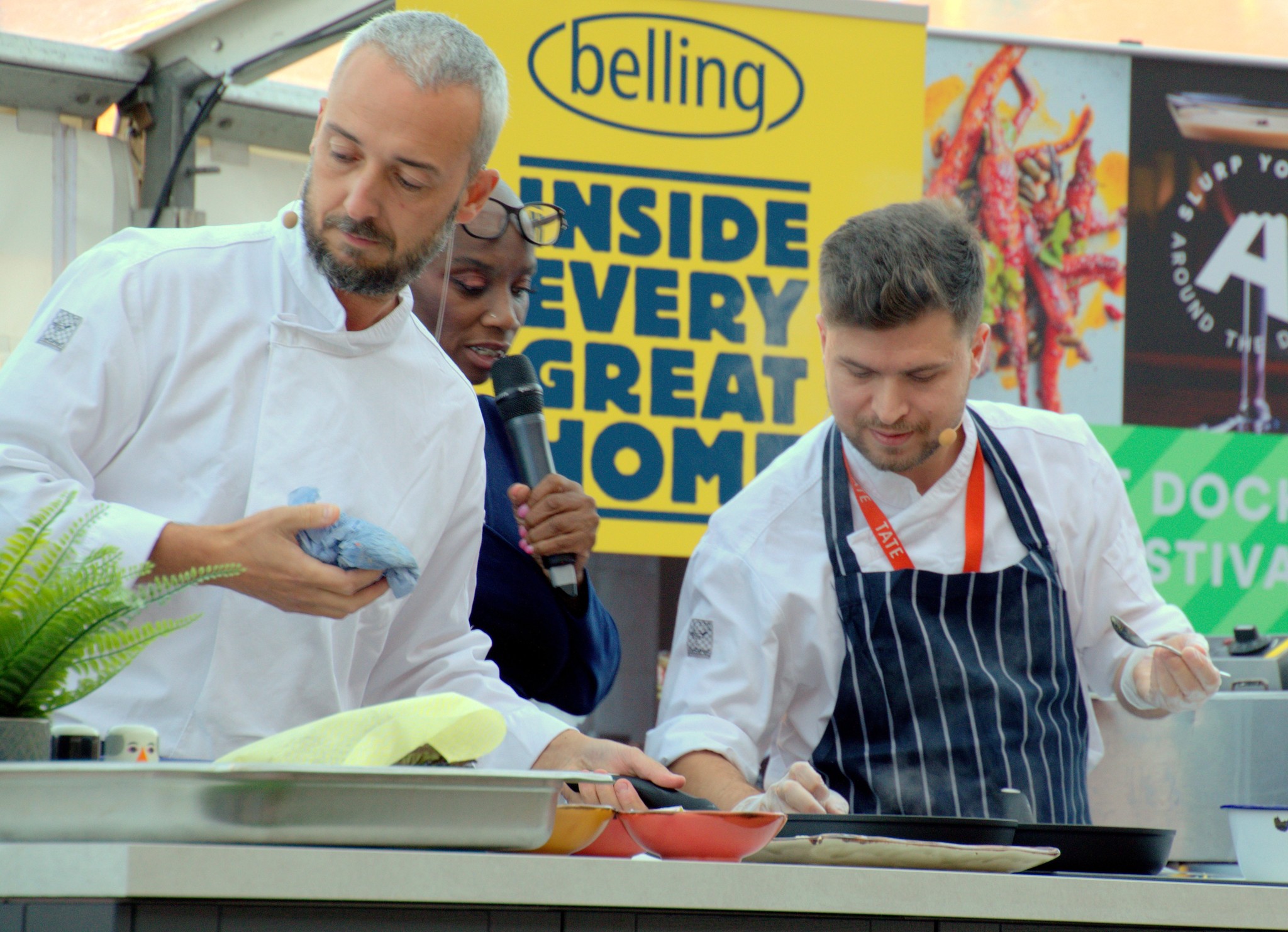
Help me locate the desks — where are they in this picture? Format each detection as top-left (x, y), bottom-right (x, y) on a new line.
top-left (0, 842), bottom-right (1288, 932)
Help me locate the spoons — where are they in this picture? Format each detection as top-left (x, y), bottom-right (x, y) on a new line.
top-left (1110, 614), bottom-right (1231, 680)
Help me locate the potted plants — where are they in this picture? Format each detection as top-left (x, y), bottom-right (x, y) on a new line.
top-left (0, 489), bottom-right (248, 757)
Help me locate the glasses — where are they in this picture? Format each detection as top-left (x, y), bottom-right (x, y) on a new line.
top-left (458, 195), bottom-right (568, 246)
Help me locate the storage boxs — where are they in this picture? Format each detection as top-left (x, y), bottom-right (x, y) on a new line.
top-left (1088, 692), bottom-right (1288, 865)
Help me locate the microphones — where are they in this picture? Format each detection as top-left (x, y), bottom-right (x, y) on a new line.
top-left (489, 355), bottom-right (580, 601)
top-left (281, 189), bottom-right (302, 229)
top-left (938, 418), bottom-right (964, 447)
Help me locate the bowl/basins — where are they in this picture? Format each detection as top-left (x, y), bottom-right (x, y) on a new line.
top-left (1221, 803), bottom-right (1288, 884)
top-left (525, 805), bottom-right (614, 855)
top-left (619, 806), bottom-right (793, 862)
top-left (580, 809), bottom-right (648, 857)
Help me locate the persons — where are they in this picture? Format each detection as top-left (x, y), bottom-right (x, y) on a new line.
top-left (0, 9), bottom-right (688, 814)
top-left (642, 199), bottom-right (1221, 816)
top-left (410, 179), bottom-right (624, 716)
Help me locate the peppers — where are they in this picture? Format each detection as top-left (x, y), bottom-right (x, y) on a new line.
top-left (928, 44), bottom-right (1127, 413)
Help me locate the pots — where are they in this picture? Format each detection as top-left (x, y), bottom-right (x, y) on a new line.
top-left (994, 787), bottom-right (1180, 876)
top-left (563, 769), bottom-right (1020, 846)
